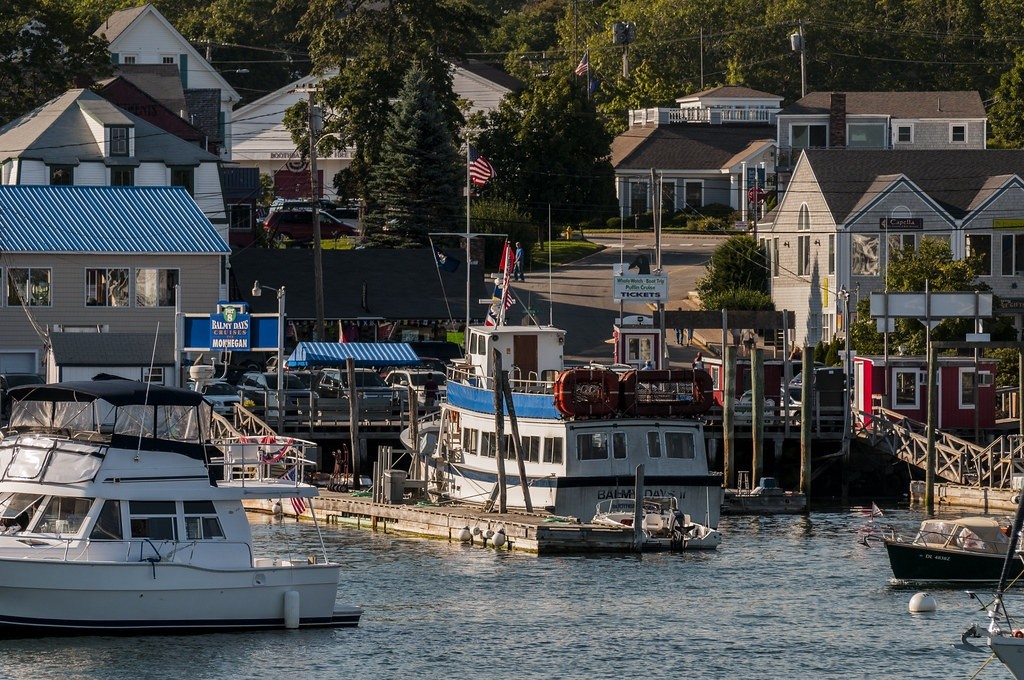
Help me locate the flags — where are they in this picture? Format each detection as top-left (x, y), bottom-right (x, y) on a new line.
top-left (469, 143), bottom-right (497, 186)
top-left (281, 467), bottom-right (305, 516)
top-left (499, 242), bottom-right (514, 273)
top-left (574, 52), bottom-right (588, 76)
top-left (484, 285), bottom-right (513, 326)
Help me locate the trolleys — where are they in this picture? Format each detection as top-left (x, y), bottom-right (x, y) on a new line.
top-left (326, 443), bottom-right (350, 493)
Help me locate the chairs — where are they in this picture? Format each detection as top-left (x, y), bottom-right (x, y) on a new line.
top-left (642, 514), bottom-right (663, 529)
top-left (227, 444), bottom-right (263, 481)
top-left (4, 510), bottom-right (29, 529)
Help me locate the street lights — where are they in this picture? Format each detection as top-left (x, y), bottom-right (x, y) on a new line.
top-left (294, 83), bottom-right (345, 342)
top-left (252, 280), bottom-right (286, 436)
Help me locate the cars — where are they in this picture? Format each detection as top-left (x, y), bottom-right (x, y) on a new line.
top-left (255, 204), bottom-right (269, 225)
top-left (269, 198), bottom-right (313, 213)
top-left (186, 340), bottom-right (466, 418)
top-left (336, 198), bottom-right (364, 218)
top-left (307, 198), bottom-right (337, 216)
top-left (263, 209), bottom-right (360, 240)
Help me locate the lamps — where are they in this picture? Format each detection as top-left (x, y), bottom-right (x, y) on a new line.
top-left (637, 316), bottom-right (643, 325)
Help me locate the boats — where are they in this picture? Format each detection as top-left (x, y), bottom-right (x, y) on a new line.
top-left (882, 517), bottom-right (1024, 589)
top-left (949, 590), bottom-right (1024, 680)
top-left (620, 369), bottom-right (713, 417)
top-left (591, 495), bottom-right (722, 551)
top-left (0, 380), bottom-right (366, 637)
top-left (721, 477), bottom-right (806, 516)
top-left (399, 138), bottom-right (724, 531)
top-left (554, 368), bottom-right (620, 418)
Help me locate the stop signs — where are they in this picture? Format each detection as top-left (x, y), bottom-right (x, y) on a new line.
top-left (747, 187), bottom-right (764, 204)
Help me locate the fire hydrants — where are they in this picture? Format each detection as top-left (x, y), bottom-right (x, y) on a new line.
top-left (566, 226), bottom-right (573, 240)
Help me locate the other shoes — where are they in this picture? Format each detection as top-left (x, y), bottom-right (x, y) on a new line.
top-left (512, 279), bottom-right (518, 282)
top-left (518, 279), bottom-right (525, 283)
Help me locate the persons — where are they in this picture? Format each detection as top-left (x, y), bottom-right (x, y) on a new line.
top-left (512, 242), bottom-right (525, 283)
top-left (730, 329), bottom-right (756, 357)
top-left (673, 307), bottom-right (694, 347)
top-left (692, 352), bottom-right (704, 369)
top-left (641, 360), bottom-right (655, 370)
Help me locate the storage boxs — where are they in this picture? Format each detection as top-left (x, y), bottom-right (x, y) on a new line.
top-left (760, 478), bottom-right (776, 488)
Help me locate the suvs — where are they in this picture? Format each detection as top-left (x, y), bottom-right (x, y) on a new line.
top-left (788, 365), bottom-right (847, 403)
